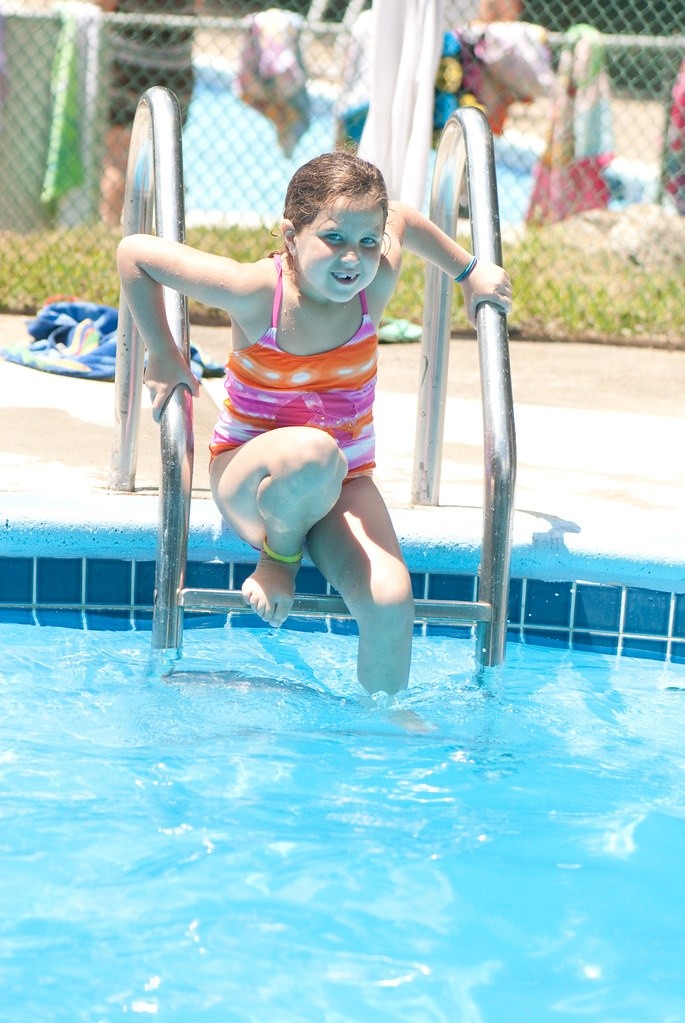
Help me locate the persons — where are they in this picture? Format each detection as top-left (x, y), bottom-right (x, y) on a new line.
top-left (117, 153), bottom-right (513, 695)
top-left (99, 0), bottom-right (194, 225)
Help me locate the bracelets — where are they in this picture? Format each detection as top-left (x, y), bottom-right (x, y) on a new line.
top-left (454, 256), bottom-right (477, 282)
